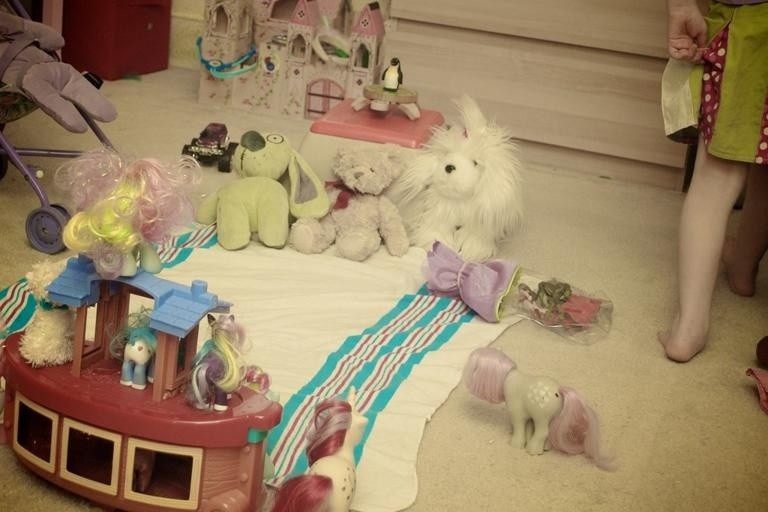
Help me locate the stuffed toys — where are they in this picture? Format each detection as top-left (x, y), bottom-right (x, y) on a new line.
top-left (20, 147), bottom-right (205, 369)
top-left (182, 123), bottom-right (239, 173)
top-left (186, 312), bottom-right (253, 412)
top-left (0, 11), bottom-right (117, 133)
top-left (468, 350), bottom-right (613, 470)
top-left (288, 146), bottom-right (410, 260)
top-left (108, 306), bottom-right (156, 390)
top-left (274, 382), bottom-right (368, 512)
top-left (399, 101), bottom-right (526, 260)
top-left (194, 128), bottom-right (329, 252)
top-left (349, 55), bottom-right (422, 122)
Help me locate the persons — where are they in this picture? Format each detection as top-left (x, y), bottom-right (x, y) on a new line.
top-left (654, 0), bottom-right (768, 363)
